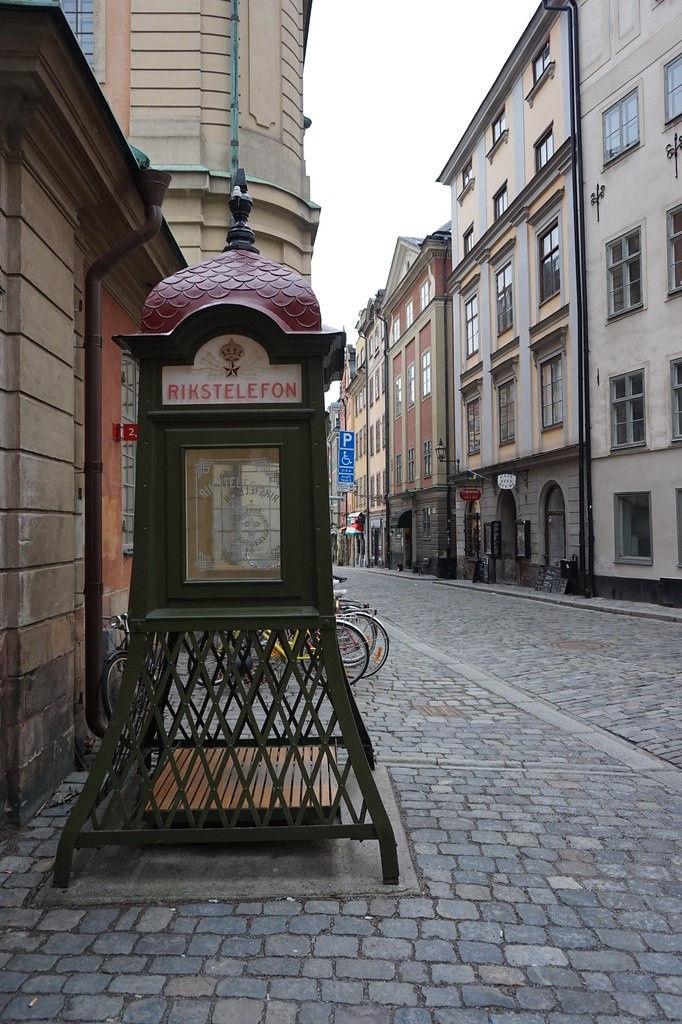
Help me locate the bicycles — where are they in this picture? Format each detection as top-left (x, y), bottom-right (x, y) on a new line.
top-left (99, 576), bottom-right (390, 751)
